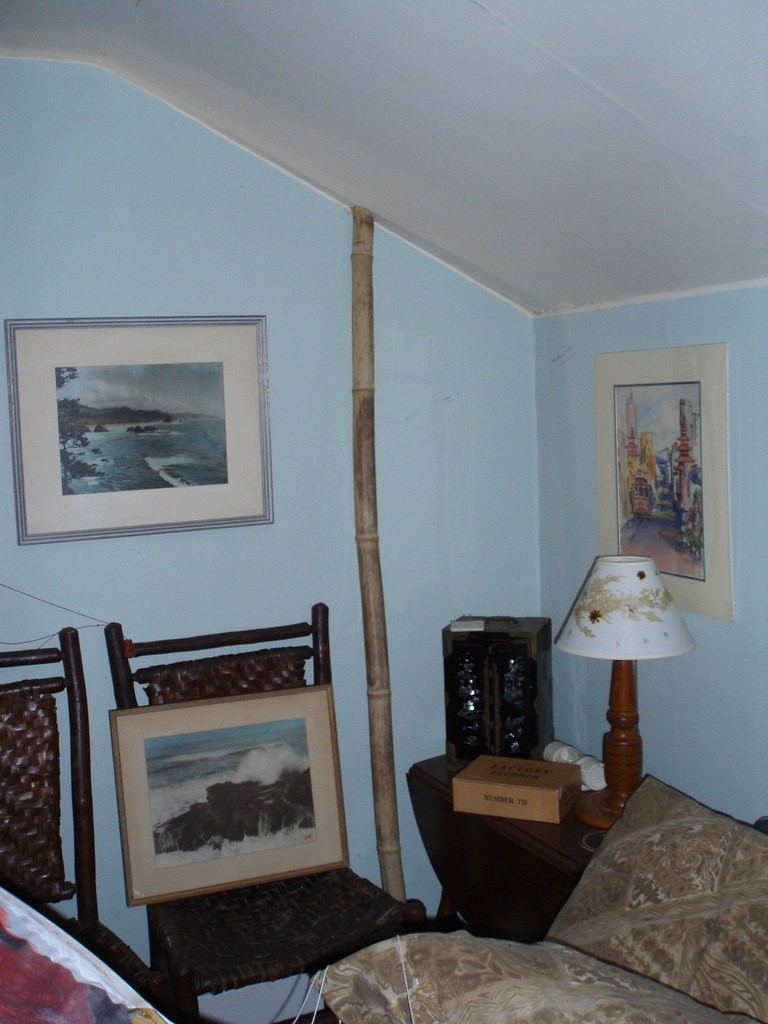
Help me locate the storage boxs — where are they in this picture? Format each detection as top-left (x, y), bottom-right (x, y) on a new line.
top-left (451, 755), bottom-right (584, 826)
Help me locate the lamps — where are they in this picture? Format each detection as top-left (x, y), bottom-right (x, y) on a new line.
top-left (554, 555), bottom-right (694, 828)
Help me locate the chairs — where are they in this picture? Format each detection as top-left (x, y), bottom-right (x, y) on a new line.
top-left (103, 602), bottom-right (426, 1024)
top-left (0, 626), bottom-right (203, 1024)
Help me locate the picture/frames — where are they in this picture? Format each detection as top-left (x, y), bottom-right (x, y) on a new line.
top-left (4, 314), bottom-right (273, 548)
top-left (594, 341), bottom-right (736, 620)
top-left (109, 684), bottom-right (353, 908)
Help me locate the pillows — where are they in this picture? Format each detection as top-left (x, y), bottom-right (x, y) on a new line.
top-left (311, 939), bottom-right (768, 1024)
top-left (547, 772), bottom-right (768, 1024)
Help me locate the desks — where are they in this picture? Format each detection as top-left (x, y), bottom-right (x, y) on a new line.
top-left (406, 737), bottom-right (642, 944)
